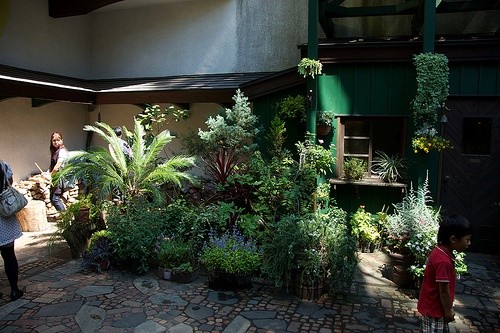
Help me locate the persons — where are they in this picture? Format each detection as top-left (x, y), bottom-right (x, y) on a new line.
top-left (41, 132), bottom-right (70, 222)
top-left (418, 214), bottom-right (471, 333)
top-left (108, 126), bottom-right (134, 205)
top-left (0, 158), bottom-right (25, 302)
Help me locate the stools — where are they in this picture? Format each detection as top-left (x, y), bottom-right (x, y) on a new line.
top-left (16, 200), bottom-right (49, 232)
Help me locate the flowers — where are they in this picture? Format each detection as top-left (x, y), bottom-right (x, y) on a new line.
top-left (412, 126), bottom-right (455, 154)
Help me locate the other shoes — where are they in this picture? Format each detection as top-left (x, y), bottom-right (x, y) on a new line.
top-left (10, 290), bottom-right (23, 300)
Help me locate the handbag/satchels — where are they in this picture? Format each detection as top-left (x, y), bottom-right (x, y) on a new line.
top-left (0, 187), bottom-right (28, 218)
top-left (59, 168), bottom-right (75, 191)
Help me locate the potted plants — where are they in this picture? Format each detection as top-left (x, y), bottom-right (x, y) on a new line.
top-left (45, 51), bottom-right (469, 302)
